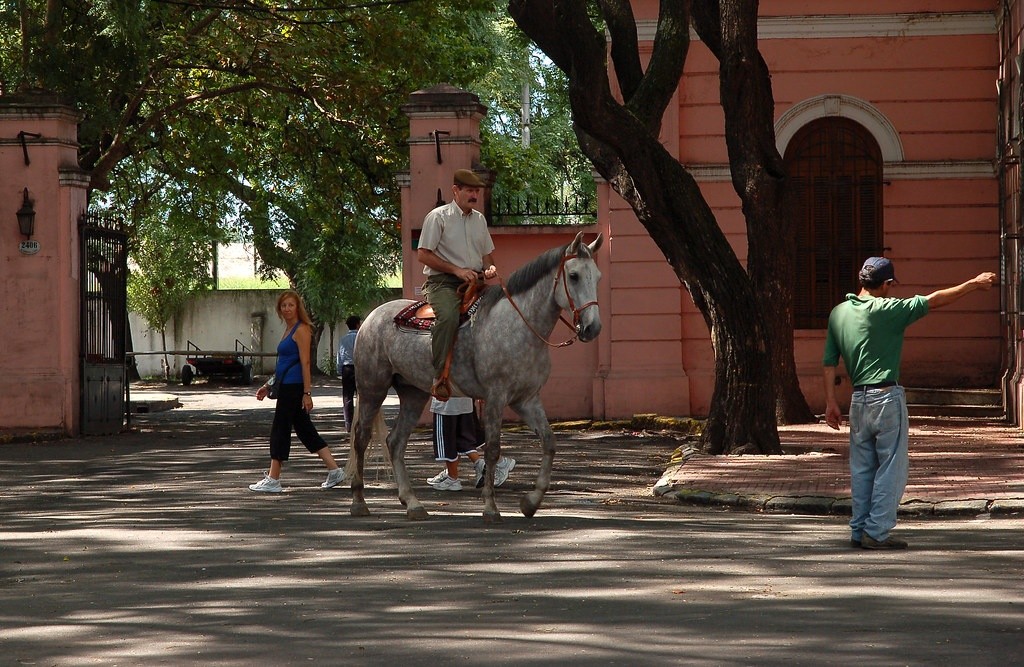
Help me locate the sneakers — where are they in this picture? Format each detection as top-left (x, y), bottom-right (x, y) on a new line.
top-left (852, 540), bottom-right (860, 548)
top-left (432, 477), bottom-right (463, 491)
top-left (427, 470), bottom-right (449, 485)
top-left (321, 467), bottom-right (347, 488)
top-left (494, 455), bottom-right (516, 487)
top-left (860, 534), bottom-right (908, 549)
top-left (248, 471), bottom-right (282, 493)
top-left (474, 460), bottom-right (487, 489)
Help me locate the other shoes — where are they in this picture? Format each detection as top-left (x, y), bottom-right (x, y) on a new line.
top-left (433, 377), bottom-right (449, 402)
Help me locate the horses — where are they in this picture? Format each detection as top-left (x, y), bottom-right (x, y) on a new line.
top-left (343, 229), bottom-right (605, 527)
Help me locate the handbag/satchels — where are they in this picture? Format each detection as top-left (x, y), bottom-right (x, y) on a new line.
top-left (266, 376), bottom-right (284, 399)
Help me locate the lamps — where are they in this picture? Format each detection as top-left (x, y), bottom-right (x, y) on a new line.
top-left (15, 187), bottom-right (37, 240)
top-left (436, 188), bottom-right (446, 208)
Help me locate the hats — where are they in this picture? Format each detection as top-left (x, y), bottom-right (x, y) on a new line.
top-left (454, 169), bottom-right (486, 188)
top-left (861, 256), bottom-right (899, 285)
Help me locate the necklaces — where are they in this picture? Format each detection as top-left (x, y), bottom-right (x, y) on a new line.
top-left (286, 324), bottom-right (294, 331)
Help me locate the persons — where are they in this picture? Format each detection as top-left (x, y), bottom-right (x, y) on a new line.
top-left (416, 169), bottom-right (499, 402)
top-left (248, 290), bottom-right (346, 493)
top-left (425, 395), bottom-right (515, 491)
top-left (822, 255), bottom-right (998, 549)
top-left (335, 315), bottom-right (362, 443)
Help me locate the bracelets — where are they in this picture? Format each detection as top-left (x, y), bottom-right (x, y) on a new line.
top-left (304, 392), bottom-right (311, 396)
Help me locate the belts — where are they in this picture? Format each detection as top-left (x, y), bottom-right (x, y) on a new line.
top-left (853, 381), bottom-right (899, 391)
